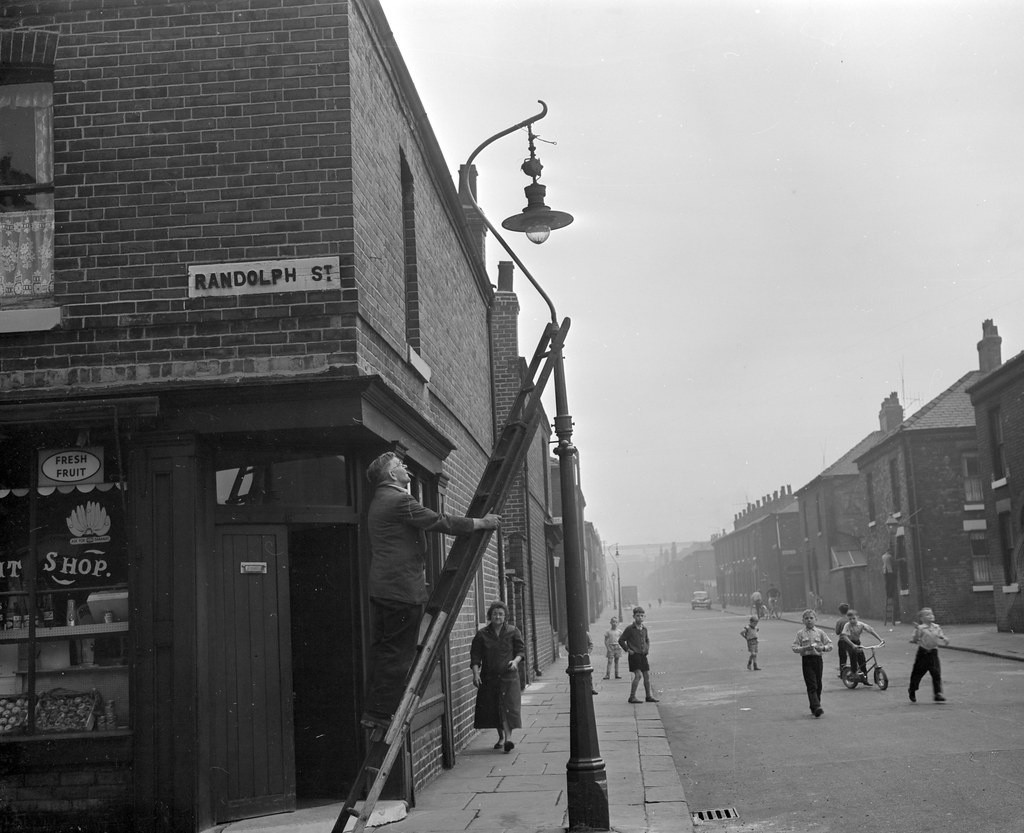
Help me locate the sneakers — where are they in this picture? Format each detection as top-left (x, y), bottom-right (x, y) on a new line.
top-left (908, 688), bottom-right (916, 702)
top-left (935, 694), bottom-right (946, 701)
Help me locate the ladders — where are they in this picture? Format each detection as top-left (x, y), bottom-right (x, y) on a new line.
top-left (329, 316), bottom-right (572, 833)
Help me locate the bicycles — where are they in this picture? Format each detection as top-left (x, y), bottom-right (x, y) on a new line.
top-left (841, 641), bottom-right (888, 690)
top-left (750, 598), bottom-right (782, 620)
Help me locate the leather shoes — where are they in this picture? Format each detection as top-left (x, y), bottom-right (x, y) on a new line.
top-left (360, 714), bottom-right (391, 730)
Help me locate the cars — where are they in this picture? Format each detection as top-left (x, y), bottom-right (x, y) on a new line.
top-left (691, 591), bottom-right (712, 610)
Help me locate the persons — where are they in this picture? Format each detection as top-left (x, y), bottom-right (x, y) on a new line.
top-left (469, 601), bottom-right (526, 752)
top-left (603, 616), bottom-right (624, 679)
top-left (740, 617), bottom-right (761, 670)
top-left (882, 549), bottom-right (905, 597)
top-left (751, 589), bottom-right (763, 619)
top-left (765, 584), bottom-right (780, 609)
top-left (360, 451), bottom-right (503, 729)
top-left (617, 606), bottom-right (660, 703)
top-left (792, 610), bottom-right (833, 717)
top-left (835, 604), bottom-right (849, 678)
top-left (564, 630), bottom-right (598, 695)
top-left (841, 609), bottom-right (885, 686)
top-left (907, 607), bottom-right (949, 702)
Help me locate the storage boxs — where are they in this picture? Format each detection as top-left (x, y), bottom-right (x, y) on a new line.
top-left (0, 688), bottom-right (102, 737)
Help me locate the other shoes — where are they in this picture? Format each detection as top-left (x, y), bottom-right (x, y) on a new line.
top-left (628, 697), bottom-right (643, 703)
top-left (504, 741), bottom-right (514, 752)
top-left (754, 667), bottom-right (761, 670)
top-left (646, 696), bottom-right (660, 703)
top-left (815, 708), bottom-right (824, 718)
top-left (493, 742), bottom-right (503, 749)
top-left (616, 676), bottom-right (622, 679)
top-left (747, 665), bottom-right (751, 670)
top-left (864, 680), bottom-right (873, 686)
top-left (604, 676), bottom-right (610, 679)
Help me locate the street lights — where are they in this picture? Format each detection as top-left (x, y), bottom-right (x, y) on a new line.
top-left (608, 543), bottom-right (623, 622)
top-left (612, 573), bottom-right (616, 608)
top-left (466, 101), bottom-right (611, 832)
top-left (885, 512), bottom-right (902, 625)
